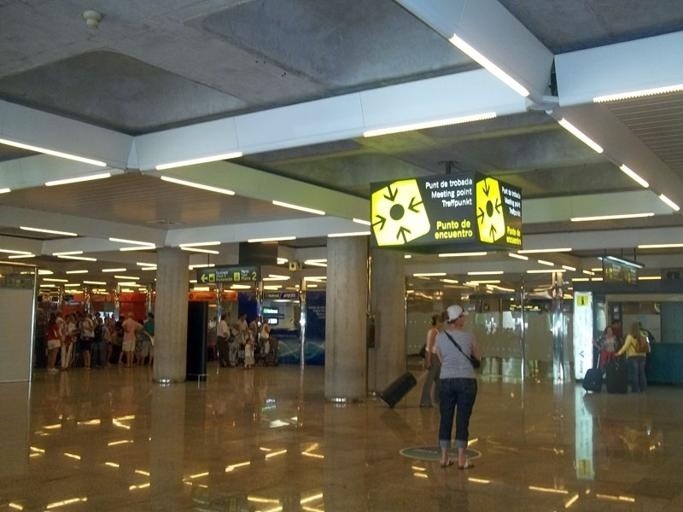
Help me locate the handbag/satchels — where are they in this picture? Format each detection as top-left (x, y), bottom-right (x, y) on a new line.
top-left (420, 344), bottom-right (426, 357)
top-left (471, 355), bottom-right (480, 368)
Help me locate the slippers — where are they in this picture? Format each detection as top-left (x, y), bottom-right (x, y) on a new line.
top-left (458, 460), bottom-right (474, 469)
top-left (441, 460), bottom-right (453, 468)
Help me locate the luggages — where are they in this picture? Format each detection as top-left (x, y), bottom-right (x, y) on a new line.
top-left (380, 369), bottom-right (428, 407)
top-left (606, 357), bottom-right (628, 393)
top-left (582, 352), bottom-right (601, 393)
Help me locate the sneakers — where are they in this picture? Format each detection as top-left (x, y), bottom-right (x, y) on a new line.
top-left (48, 368), bottom-right (57, 372)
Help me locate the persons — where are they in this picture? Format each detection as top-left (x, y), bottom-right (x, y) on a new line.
top-left (614, 322), bottom-right (651, 392)
top-left (45, 367), bottom-right (269, 420)
top-left (602, 318), bottom-right (625, 347)
top-left (435, 305), bottom-right (483, 469)
top-left (637, 321), bottom-right (655, 384)
top-left (438, 468), bottom-right (470, 512)
top-left (217, 310), bottom-right (271, 370)
top-left (418, 313), bottom-right (448, 407)
top-left (591, 326), bottom-right (620, 378)
top-left (45, 310), bottom-right (154, 371)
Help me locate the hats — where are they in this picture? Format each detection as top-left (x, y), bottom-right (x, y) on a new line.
top-left (445, 305), bottom-right (463, 322)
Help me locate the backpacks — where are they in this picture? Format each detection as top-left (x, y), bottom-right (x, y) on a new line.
top-left (630, 332), bottom-right (651, 353)
